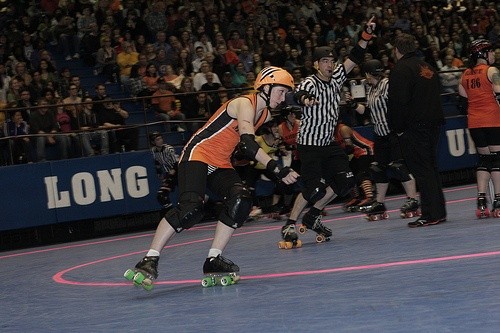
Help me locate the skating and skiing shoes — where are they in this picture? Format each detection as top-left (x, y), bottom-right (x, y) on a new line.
top-left (201, 253), bottom-right (240, 288)
top-left (492, 193), bottom-right (500, 218)
top-left (277, 223), bottom-right (302, 249)
top-left (123, 255), bottom-right (160, 291)
top-left (245, 204), bottom-right (329, 222)
top-left (365, 202), bottom-right (390, 222)
top-left (299, 212), bottom-right (332, 243)
top-left (343, 195), bottom-right (376, 214)
top-left (475, 191), bottom-right (490, 218)
top-left (400, 197), bottom-right (422, 218)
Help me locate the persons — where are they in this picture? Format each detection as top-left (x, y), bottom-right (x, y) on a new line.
top-left (124, 66), bottom-right (302, 289)
top-left (380, 34), bottom-right (448, 227)
top-left (276, 16), bottom-right (377, 249)
top-left (457, 39), bottom-right (499, 219)
top-left (0, 0), bottom-right (500, 219)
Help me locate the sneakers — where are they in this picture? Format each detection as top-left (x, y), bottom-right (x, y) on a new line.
top-left (407, 215), bottom-right (447, 227)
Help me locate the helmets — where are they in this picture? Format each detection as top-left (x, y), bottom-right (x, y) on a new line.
top-left (280, 106), bottom-right (295, 118)
top-left (253, 66), bottom-right (295, 94)
top-left (469, 38), bottom-right (493, 54)
top-left (362, 59), bottom-right (385, 72)
top-left (148, 130), bottom-right (162, 142)
top-left (311, 46), bottom-right (338, 63)
